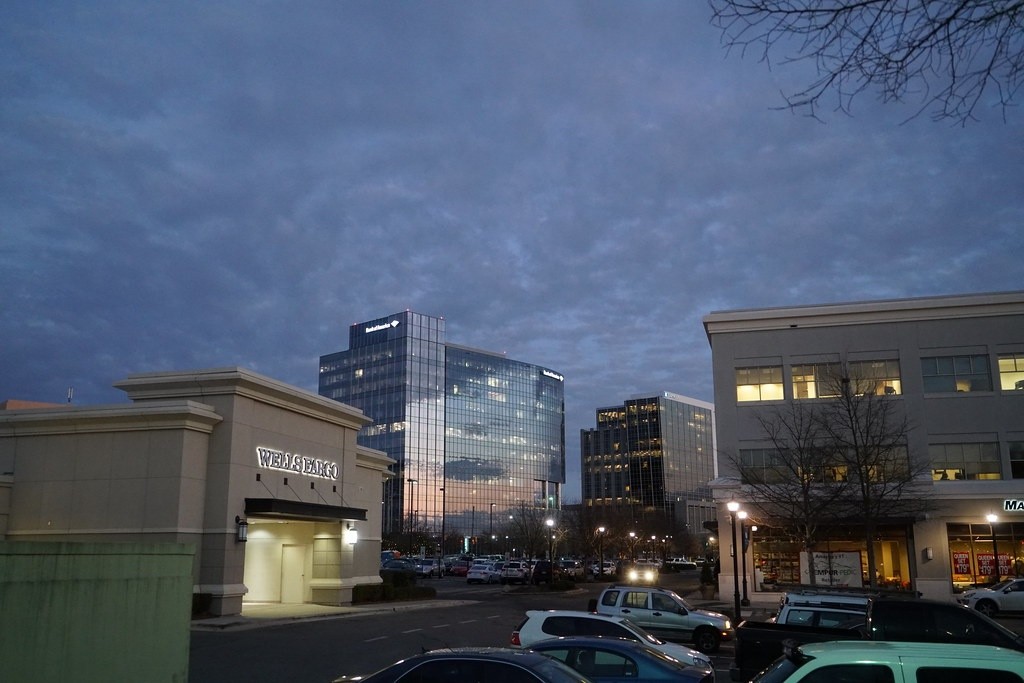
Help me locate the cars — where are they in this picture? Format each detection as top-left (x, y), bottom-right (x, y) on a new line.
top-left (330, 609), bottom-right (717, 683)
top-left (382, 549), bottom-right (705, 584)
top-left (748, 640), bottom-right (1023, 682)
top-left (956, 577), bottom-right (1024, 617)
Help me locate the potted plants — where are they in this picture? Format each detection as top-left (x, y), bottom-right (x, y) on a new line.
top-left (698, 561), bottom-right (716, 600)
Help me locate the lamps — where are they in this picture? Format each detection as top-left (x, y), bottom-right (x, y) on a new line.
top-left (349, 528), bottom-right (358, 544)
top-left (235, 515), bottom-right (249, 542)
top-left (332, 486), bottom-right (350, 507)
top-left (257, 474), bottom-right (274, 499)
top-left (310, 482), bottom-right (328, 504)
top-left (283, 477), bottom-right (303, 502)
top-left (730, 544), bottom-right (734, 556)
top-left (926, 547), bottom-right (933, 561)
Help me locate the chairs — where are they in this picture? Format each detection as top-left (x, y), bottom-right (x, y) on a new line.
top-left (1015, 380), bottom-right (1024, 389)
top-left (885, 386), bottom-right (896, 395)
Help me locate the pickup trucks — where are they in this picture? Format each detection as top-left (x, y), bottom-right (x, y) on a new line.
top-left (726, 597), bottom-right (1023, 680)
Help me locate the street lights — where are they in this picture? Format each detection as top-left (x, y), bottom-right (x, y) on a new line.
top-left (727, 496), bottom-right (741, 629)
top-left (630, 531), bottom-right (635, 568)
top-left (651, 535), bottom-right (656, 563)
top-left (490, 502), bottom-right (496, 554)
top-left (547, 519), bottom-right (554, 585)
top-left (407, 478), bottom-right (417, 558)
top-left (440, 488), bottom-right (446, 556)
top-left (598, 524), bottom-right (605, 580)
top-left (647, 538), bottom-right (650, 552)
top-left (987, 513), bottom-right (1000, 583)
top-left (738, 510), bottom-right (750, 606)
top-left (415, 510), bottom-right (418, 556)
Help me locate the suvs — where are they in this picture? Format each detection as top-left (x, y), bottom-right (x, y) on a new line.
top-left (765, 583), bottom-right (918, 633)
top-left (587, 585), bottom-right (736, 654)
